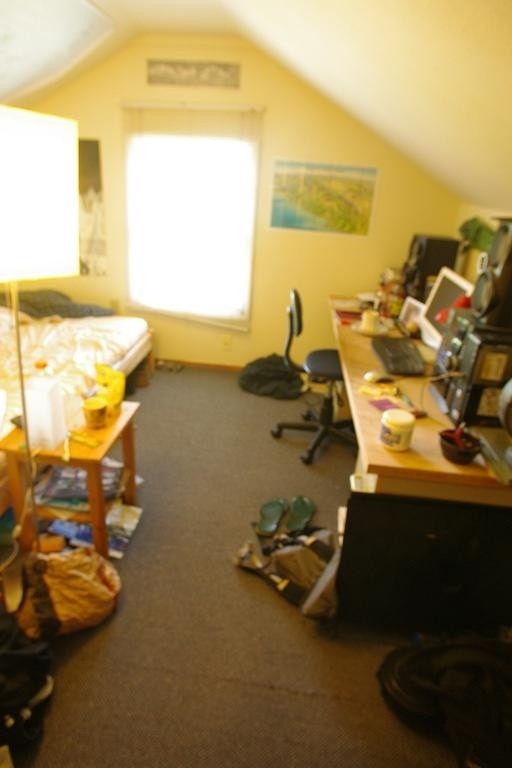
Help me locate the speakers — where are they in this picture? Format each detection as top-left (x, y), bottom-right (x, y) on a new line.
top-left (470, 219), bottom-right (512, 345)
top-left (406, 234), bottom-right (459, 276)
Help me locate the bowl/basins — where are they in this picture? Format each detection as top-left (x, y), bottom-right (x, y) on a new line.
top-left (438, 428), bottom-right (484, 465)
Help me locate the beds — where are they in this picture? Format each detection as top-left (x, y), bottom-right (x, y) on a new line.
top-left (0, 312), bottom-right (155, 518)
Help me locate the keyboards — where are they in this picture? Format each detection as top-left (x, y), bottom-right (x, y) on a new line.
top-left (373, 337), bottom-right (425, 375)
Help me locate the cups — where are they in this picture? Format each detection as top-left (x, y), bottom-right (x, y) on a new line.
top-left (361, 309), bottom-right (380, 331)
top-left (81, 398), bottom-right (107, 428)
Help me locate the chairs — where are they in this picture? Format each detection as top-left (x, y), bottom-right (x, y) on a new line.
top-left (269, 287), bottom-right (358, 465)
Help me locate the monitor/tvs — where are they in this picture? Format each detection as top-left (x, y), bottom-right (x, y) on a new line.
top-left (418, 266), bottom-right (475, 349)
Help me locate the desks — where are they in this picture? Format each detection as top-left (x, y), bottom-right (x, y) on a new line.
top-left (327, 293), bottom-right (511, 627)
top-left (0, 400), bottom-right (142, 562)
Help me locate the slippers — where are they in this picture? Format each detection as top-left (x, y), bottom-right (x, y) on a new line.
top-left (255, 497), bottom-right (289, 536)
top-left (285, 495), bottom-right (315, 534)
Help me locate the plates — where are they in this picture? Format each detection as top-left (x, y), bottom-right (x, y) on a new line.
top-left (351, 322), bottom-right (389, 338)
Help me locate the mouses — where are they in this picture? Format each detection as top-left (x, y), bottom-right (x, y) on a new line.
top-left (364, 371), bottom-right (394, 385)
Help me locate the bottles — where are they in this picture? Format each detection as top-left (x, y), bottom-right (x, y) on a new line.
top-left (22, 361), bottom-right (67, 450)
top-left (379, 409), bottom-right (416, 452)
